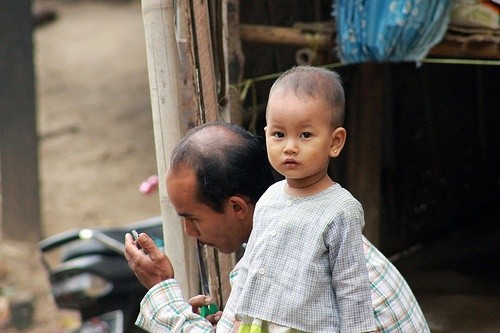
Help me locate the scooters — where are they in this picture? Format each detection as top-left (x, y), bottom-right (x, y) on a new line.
top-left (35, 172), bottom-right (183, 333)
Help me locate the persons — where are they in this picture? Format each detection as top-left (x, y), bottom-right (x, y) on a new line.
top-left (235, 65), bottom-right (376, 333)
top-left (122, 120), bottom-right (431, 333)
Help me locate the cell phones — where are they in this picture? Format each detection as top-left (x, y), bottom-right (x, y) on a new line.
top-left (131, 230), bottom-right (142, 250)
